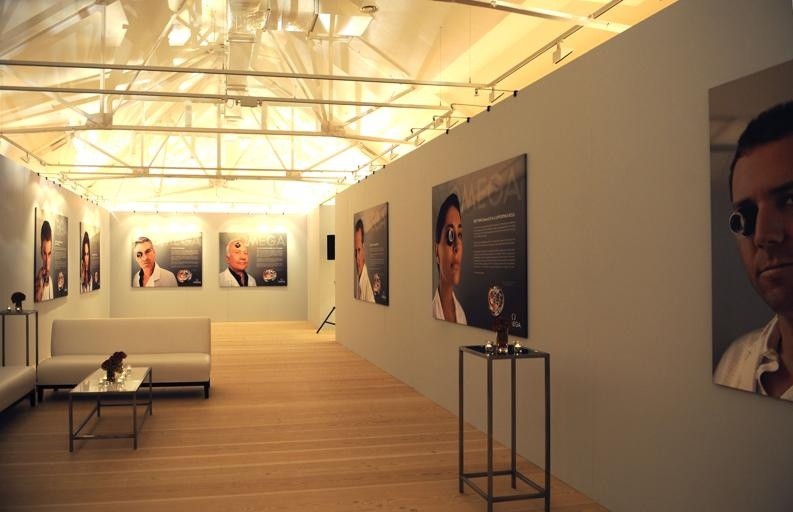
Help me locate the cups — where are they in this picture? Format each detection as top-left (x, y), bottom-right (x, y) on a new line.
top-left (81, 363), bottom-right (133, 391)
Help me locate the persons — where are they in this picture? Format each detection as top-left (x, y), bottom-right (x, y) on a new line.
top-left (36, 220), bottom-right (54, 301)
top-left (354, 219), bottom-right (376, 302)
top-left (131, 236), bottom-right (178, 286)
top-left (431, 192), bottom-right (468, 324)
top-left (80, 231), bottom-right (93, 293)
top-left (218, 238), bottom-right (257, 287)
top-left (713, 99), bottom-right (793, 401)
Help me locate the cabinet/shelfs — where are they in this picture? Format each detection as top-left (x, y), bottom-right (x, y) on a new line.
top-left (458, 346), bottom-right (551, 512)
top-left (0, 310), bottom-right (38, 365)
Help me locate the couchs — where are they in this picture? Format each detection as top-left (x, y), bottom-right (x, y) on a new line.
top-left (36, 317), bottom-right (211, 401)
top-left (0, 365), bottom-right (36, 413)
